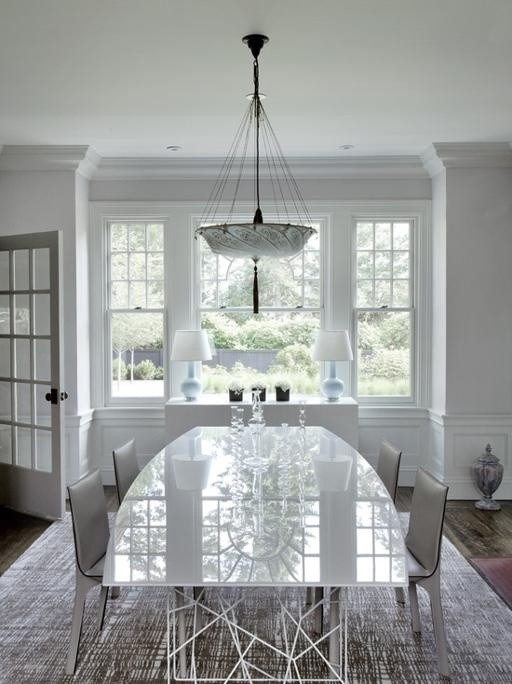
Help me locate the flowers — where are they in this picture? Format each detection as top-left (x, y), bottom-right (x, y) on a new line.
top-left (226, 372), bottom-right (292, 395)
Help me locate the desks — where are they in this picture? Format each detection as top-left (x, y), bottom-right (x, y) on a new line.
top-left (164, 393), bottom-right (358, 444)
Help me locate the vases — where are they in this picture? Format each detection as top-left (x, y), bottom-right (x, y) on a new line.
top-left (252, 388), bottom-right (266, 402)
top-left (277, 387), bottom-right (289, 401)
top-left (229, 389), bottom-right (242, 401)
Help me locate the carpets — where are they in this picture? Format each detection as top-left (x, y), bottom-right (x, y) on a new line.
top-left (0, 512), bottom-right (512, 684)
top-left (471, 558), bottom-right (512, 609)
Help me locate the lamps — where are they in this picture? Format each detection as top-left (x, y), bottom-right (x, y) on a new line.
top-left (311, 426), bottom-right (354, 492)
top-left (311, 330), bottom-right (354, 402)
top-left (170, 328), bottom-right (213, 402)
top-left (194, 34), bottom-right (319, 314)
top-left (171, 426), bottom-right (213, 492)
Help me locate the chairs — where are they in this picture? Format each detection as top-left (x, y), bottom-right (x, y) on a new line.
top-left (314, 465), bottom-right (450, 680)
top-left (65, 465), bottom-right (203, 679)
top-left (306, 440), bottom-right (406, 605)
top-left (111, 438), bottom-right (204, 599)
